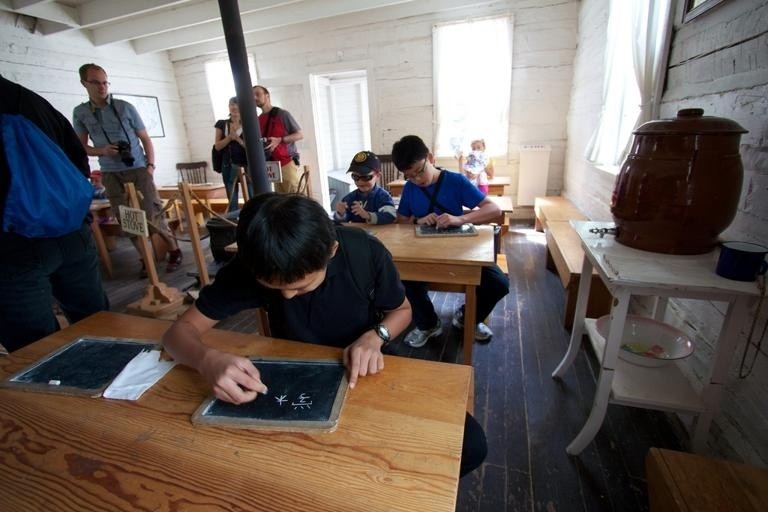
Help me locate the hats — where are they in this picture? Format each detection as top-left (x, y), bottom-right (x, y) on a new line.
top-left (344, 151), bottom-right (381, 176)
top-left (88, 170), bottom-right (104, 177)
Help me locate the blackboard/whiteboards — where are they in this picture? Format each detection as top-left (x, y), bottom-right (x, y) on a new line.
top-left (414, 223), bottom-right (478, 236)
top-left (2, 333), bottom-right (161, 399)
top-left (192, 354), bottom-right (351, 434)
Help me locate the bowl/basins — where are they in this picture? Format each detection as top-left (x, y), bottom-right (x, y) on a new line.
top-left (595, 314), bottom-right (694, 368)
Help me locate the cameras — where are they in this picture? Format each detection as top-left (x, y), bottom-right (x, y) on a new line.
top-left (112, 141), bottom-right (136, 167)
top-left (260, 137), bottom-right (274, 163)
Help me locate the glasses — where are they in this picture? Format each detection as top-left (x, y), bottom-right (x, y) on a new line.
top-left (350, 172), bottom-right (378, 181)
top-left (82, 78), bottom-right (112, 88)
top-left (404, 157), bottom-right (427, 179)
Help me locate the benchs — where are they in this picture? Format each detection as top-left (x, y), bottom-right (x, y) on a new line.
top-left (531, 192), bottom-right (613, 334)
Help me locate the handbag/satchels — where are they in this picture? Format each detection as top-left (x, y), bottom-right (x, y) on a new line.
top-left (212, 120), bottom-right (226, 173)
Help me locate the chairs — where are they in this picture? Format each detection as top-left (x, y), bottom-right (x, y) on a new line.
top-left (170, 160), bottom-right (215, 232)
top-left (372, 153), bottom-right (407, 196)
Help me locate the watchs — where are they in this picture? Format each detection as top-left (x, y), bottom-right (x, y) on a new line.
top-left (373, 324), bottom-right (391, 345)
top-left (146, 163), bottom-right (158, 171)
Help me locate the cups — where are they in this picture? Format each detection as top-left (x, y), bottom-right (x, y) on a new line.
top-left (715, 239), bottom-right (767, 281)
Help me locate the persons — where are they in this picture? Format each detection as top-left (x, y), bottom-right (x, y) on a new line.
top-left (0, 74), bottom-right (111, 353)
top-left (251, 85), bottom-right (305, 195)
top-left (162, 192), bottom-right (488, 482)
top-left (73, 63), bottom-right (183, 280)
top-left (89, 168), bottom-right (113, 228)
top-left (333, 150), bottom-right (398, 225)
top-left (391, 135), bottom-right (510, 349)
top-left (215, 97), bottom-right (248, 211)
top-left (457, 140), bottom-right (494, 196)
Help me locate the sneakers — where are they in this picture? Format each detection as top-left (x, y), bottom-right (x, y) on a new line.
top-left (450, 304), bottom-right (492, 342)
top-left (403, 318), bottom-right (445, 348)
top-left (165, 248), bottom-right (184, 273)
top-left (138, 257), bottom-right (148, 279)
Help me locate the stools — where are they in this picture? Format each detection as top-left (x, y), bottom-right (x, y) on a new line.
top-left (641, 441), bottom-right (767, 512)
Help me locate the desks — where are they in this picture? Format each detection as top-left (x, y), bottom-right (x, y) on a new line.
top-left (223, 213), bottom-right (495, 366)
top-left (386, 175), bottom-right (515, 198)
top-left (1, 308), bottom-right (474, 512)
top-left (389, 191), bottom-right (513, 251)
top-left (548, 218), bottom-right (762, 458)
top-left (87, 192), bottom-right (150, 282)
top-left (155, 178), bottom-right (227, 231)
